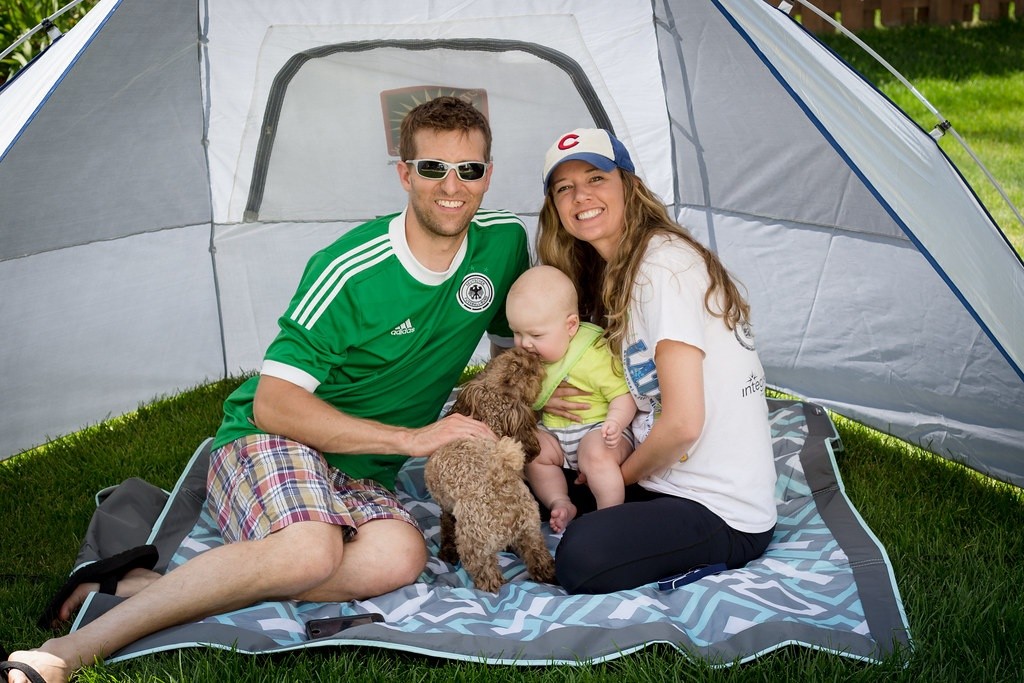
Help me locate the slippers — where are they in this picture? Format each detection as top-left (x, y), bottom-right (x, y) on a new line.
top-left (1, 643), bottom-right (48, 682)
top-left (37, 543), bottom-right (159, 631)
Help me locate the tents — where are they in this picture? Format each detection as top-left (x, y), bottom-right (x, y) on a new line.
top-left (0, 0), bottom-right (1024, 490)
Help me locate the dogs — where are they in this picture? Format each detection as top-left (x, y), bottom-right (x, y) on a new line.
top-left (423, 347), bottom-right (561, 594)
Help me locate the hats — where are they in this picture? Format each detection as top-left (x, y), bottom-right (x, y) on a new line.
top-left (543, 127), bottom-right (635, 196)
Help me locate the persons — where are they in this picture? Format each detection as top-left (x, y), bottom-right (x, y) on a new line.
top-left (502, 265), bottom-right (639, 532)
top-left (0, 96), bottom-right (534, 683)
top-left (530, 127), bottom-right (778, 599)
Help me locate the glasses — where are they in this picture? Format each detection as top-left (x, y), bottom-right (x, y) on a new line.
top-left (405, 158), bottom-right (489, 181)
